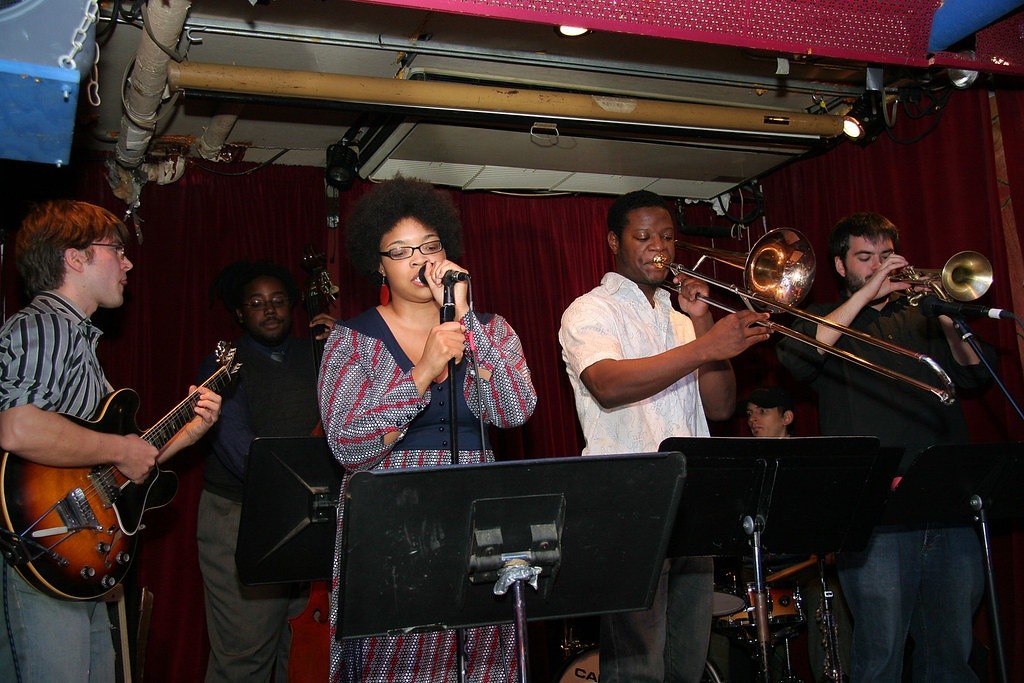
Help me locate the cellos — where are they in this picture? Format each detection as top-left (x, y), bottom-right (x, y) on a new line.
top-left (288, 242), bottom-right (351, 682)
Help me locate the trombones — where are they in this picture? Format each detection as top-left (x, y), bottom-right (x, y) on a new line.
top-left (652, 226), bottom-right (956, 409)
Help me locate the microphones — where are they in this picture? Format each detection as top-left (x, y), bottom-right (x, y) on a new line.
top-left (418, 263), bottom-right (471, 287)
top-left (918, 294), bottom-right (1016, 321)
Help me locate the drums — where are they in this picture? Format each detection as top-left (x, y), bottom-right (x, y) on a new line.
top-left (557, 631), bottom-right (723, 683)
top-left (712, 555), bottom-right (746, 617)
top-left (718, 584), bottom-right (805, 629)
top-left (759, 552), bottom-right (821, 583)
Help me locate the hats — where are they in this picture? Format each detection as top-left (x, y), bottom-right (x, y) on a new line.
top-left (739, 389), bottom-right (794, 413)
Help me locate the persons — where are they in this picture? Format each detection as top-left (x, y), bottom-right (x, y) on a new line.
top-left (314, 171), bottom-right (536, 682)
top-left (192, 274), bottom-right (338, 683)
top-left (705, 372), bottom-right (856, 683)
top-left (0, 201), bottom-right (231, 683)
top-left (558, 180), bottom-right (776, 683)
top-left (778, 207), bottom-right (1015, 682)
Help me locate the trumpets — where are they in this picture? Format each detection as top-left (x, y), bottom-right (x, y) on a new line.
top-left (888, 249), bottom-right (993, 310)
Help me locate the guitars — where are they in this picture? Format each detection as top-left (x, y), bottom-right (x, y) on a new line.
top-left (0, 341), bottom-right (244, 602)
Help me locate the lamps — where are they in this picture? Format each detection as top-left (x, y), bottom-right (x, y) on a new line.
top-left (320, 136), bottom-right (366, 194)
top-left (838, 67), bottom-right (987, 142)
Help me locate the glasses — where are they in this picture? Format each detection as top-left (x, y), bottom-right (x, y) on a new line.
top-left (87, 241), bottom-right (126, 257)
top-left (378, 240), bottom-right (443, 261)
top-left (243, 296), bottom-right (289, 312)
